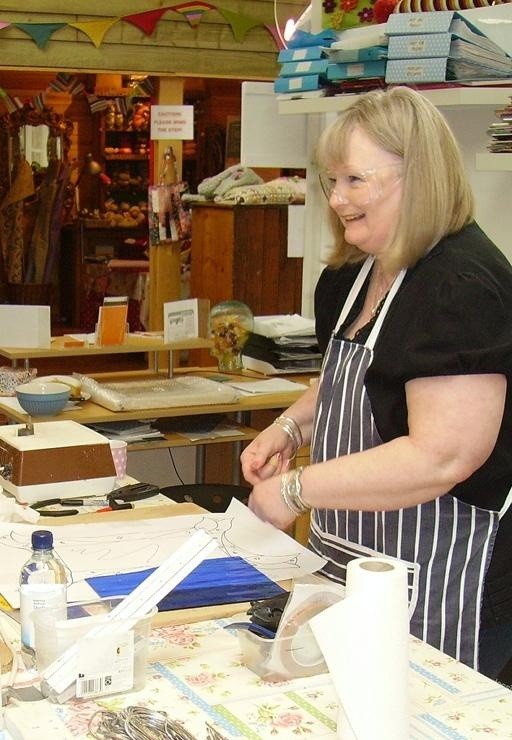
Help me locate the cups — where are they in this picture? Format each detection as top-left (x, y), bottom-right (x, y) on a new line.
top-left (108, 438), bottom-right (128, 480)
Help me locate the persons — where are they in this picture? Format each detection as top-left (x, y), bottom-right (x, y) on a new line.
top-left (240, 82), bottom-right (512, 687)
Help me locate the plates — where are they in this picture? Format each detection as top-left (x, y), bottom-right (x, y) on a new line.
top-left (65, 391), bottom-right (92, 407)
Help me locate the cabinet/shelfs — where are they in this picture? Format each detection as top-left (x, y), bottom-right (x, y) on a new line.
top-left (74, 89), bottom-right (207, 326)
top-left (0, 325), bottom-right (512, 737)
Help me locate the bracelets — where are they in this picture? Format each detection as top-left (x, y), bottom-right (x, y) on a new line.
top-left (281, 467), bottom-right (311, 517)
top-left (273, 415), bottom-right (303, 451)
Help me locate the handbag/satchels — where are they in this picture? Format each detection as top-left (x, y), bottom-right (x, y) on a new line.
top-left (149, 155), bottom-right (199, 245)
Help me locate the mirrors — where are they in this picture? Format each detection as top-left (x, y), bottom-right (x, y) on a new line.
top-left (1, 93), bottom-right (75, 205)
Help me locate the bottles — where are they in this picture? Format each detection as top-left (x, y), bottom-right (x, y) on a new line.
top-left (16, 530), bottom-right (68, 658)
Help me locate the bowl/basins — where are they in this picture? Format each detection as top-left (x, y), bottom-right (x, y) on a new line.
top-left (15, 381), bottom-right (72, 417)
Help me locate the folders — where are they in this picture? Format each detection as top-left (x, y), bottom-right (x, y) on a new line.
top-left (274, 11), bottom-right (512, 95)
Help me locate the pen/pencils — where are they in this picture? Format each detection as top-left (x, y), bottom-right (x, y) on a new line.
top-left (60, 499), bottom-right (110, 506)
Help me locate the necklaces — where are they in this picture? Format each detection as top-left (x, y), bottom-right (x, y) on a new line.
top-left (371, 264), bottom-right (398, 315)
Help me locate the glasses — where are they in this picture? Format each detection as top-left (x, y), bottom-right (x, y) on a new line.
top-left (319, 163), bottom-right (406, 207)
